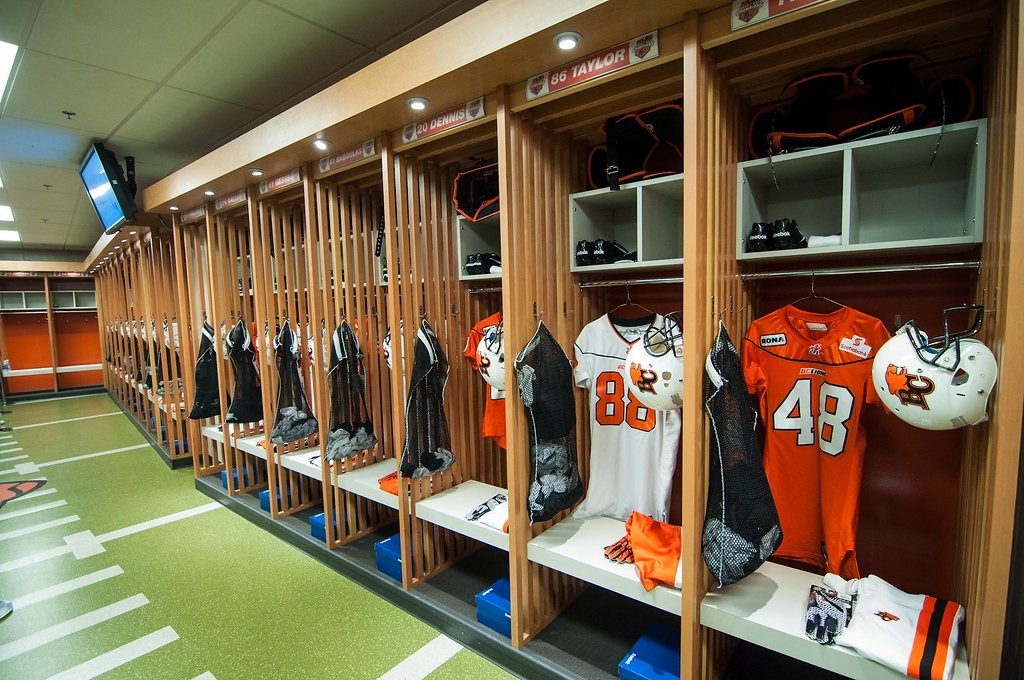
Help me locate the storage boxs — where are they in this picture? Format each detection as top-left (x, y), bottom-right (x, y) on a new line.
top-left (373, 531), bottom-right (435, 583)
top-left (221, 466), bottom-right (261, 490)
top-left (618, 615), bottom-right (680, 680)
top-left (309, 507), bottom-right (368, 544)
top-left (475, 576), bottom-right (511, 639)
top-left (259, 485), bottom-right (305, 513)
top-left (174, 438), bottom-right (189, 455)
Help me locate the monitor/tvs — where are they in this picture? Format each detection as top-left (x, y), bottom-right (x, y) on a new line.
top-left (79, 142), bottom-right (138, 236)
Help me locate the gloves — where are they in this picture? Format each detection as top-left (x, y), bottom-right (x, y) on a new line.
top-left (806, 586), bottom-right (852, 646)
top-left (604, 533), bottom-right (634, 565)
top-left (465, 493), bottom-right (507, 521)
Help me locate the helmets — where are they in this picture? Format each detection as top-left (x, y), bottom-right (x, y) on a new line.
top-left (476, 327), bottom-right (506, 390)
top-left (872, 326), bottom-right (997, 430)
top-left (625, 326), bottom-right (684, 412)
top-left (382, 319), bottom-right (405, 373)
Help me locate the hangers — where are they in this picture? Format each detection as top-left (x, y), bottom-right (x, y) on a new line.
top-left (610, 279), bottom-right (654, 314)
top-left (787, 269), bottom-right (845, 309)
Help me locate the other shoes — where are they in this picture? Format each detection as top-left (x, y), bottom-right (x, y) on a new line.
top-left (466, 253), bottom-right (501, 274)
top-left (749, 218), bottom-right (808, 251)
top-left (576, 239), bottom-right (629, 265)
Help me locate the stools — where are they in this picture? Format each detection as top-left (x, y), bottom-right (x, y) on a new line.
top-left (110, 363), bottom-right (969, 680)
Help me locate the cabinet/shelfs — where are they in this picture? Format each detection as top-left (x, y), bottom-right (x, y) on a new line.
top-left (0, 291), bottom-right (47, 310)
top-left (51, 290), bottom-right (97, 309)
top-left (233, 117), bottom-right (986, 296)
